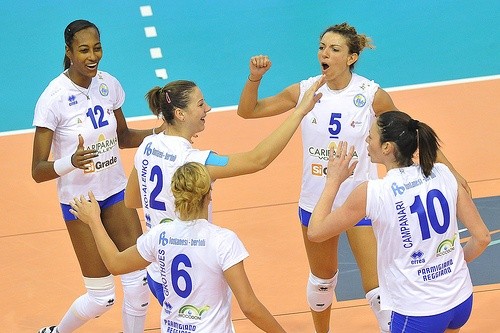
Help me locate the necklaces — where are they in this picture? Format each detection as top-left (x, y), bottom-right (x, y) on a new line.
top-left (68, 71), bottom-right (93, 100)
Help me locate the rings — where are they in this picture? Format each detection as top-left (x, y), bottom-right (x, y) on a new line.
top-left (336, 154), bottom-right (340, 158)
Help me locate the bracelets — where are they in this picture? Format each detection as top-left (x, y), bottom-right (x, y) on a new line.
top-left (248, 75), bottom-right (263, 83)
top-left (151, 127), bottom-right (156, 136)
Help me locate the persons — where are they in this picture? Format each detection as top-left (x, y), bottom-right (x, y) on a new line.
top-left (306, 112), bottom-right (492, 333)
top-left (237, 23), bottom-right (471, 333)
top-left (124, 73), bottom-right (328, 306)
top-left (32, 20), bottom-right (199, 333)
top-left (69, 162), bottom-right (285, 333)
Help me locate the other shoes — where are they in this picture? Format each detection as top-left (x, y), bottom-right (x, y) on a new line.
top-left (39, 325), bottom-right (56, 333)
